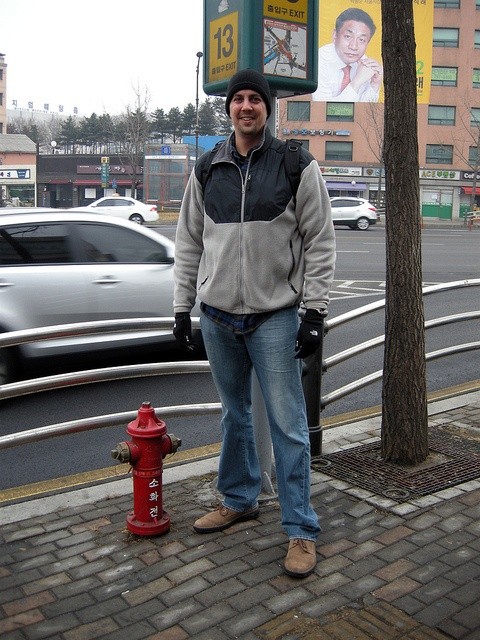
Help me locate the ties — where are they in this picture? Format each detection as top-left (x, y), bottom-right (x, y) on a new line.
top-left (340, 67), bottom-right (351, 89)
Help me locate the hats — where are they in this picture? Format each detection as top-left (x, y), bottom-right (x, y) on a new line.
top-left (225, 70), bottom-right (271, 121)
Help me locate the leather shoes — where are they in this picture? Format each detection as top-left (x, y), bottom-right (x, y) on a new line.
top-left (284, 538), bottom-right (316, 579)
top-left (194, 504), bottom-right (260, 533)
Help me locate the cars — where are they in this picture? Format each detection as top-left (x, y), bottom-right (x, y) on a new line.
top-left (76, 196), bottom-right (159, 224)
top-left (1, 213), bottom-right (199, 408)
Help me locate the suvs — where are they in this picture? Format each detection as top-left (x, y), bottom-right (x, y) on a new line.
top-left (331, 197), bottom-right (377, 231)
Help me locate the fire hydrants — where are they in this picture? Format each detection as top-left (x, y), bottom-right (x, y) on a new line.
top-left (112, 402), bottom-right (181, 536)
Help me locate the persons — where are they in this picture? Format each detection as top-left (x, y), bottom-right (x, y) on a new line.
top-left (311, 8), bottom-right (384, 102)
top-left (173, 69), bottom-right (336, 578)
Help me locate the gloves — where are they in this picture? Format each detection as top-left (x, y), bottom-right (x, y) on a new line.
top-left (173, 312), bottom-right (198, 352)
top-left (295, 308), bottom-right (327, 358)
